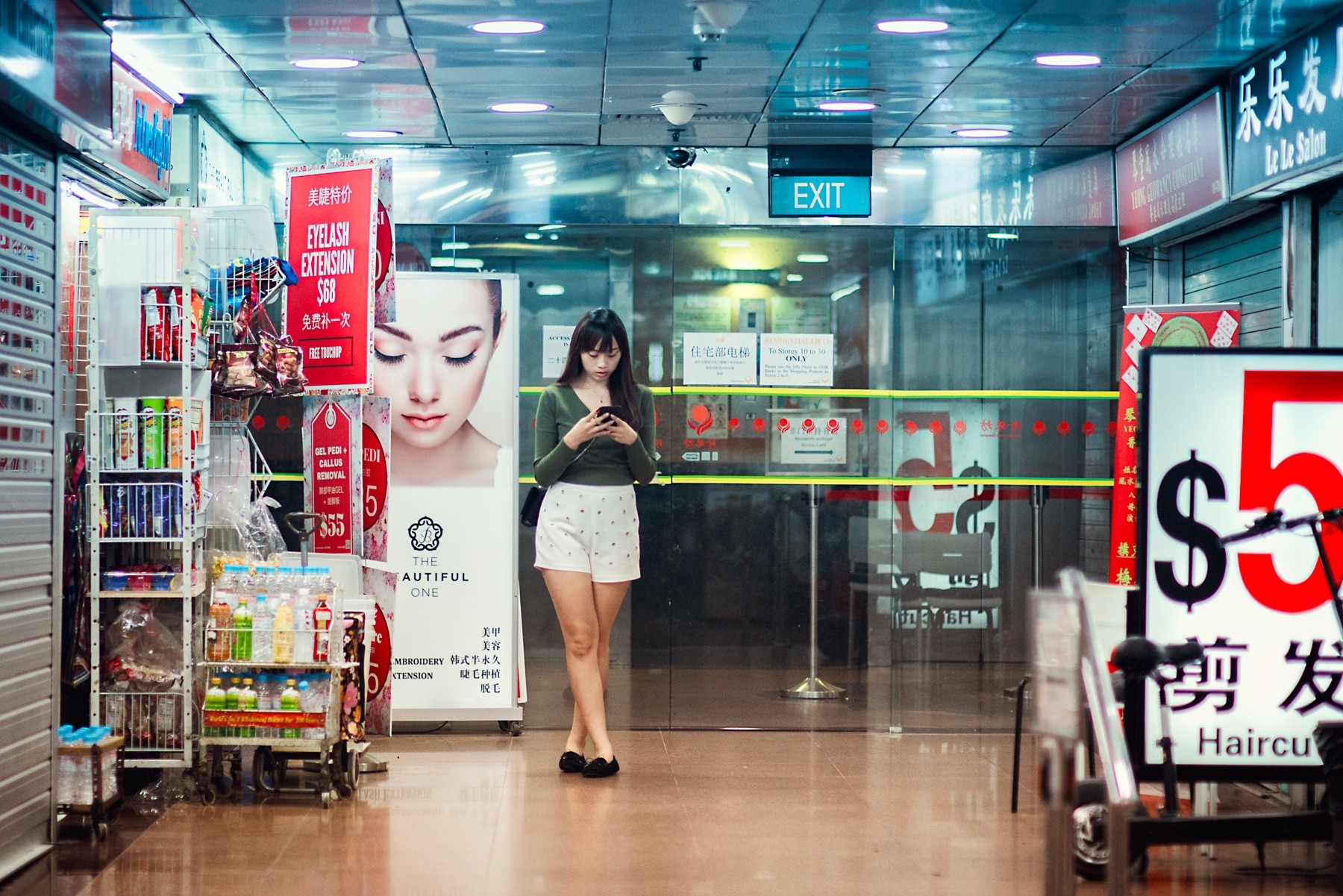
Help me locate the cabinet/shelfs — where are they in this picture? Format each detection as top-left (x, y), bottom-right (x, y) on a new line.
top-left (85, 207), bottom-right (209, 772)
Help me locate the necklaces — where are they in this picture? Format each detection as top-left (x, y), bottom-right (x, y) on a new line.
top-left (580, 375), bottom-right (606, 403)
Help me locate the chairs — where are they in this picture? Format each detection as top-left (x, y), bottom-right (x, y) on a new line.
top-left (848, 516), bottom-right (1002, 693)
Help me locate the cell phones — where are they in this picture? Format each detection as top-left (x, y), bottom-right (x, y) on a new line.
top-left (595, 405), bottom-right (622, 431)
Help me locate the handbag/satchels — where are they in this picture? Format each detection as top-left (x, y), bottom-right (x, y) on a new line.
top-left (521, 487), bottom-right (552, 528)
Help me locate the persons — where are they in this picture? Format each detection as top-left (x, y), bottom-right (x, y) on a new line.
top-left (533, 307), bottom-right (657, 778)
top-left (367, 279), bottom-right (513, 487)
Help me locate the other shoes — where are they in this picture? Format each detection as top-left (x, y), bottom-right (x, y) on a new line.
top-left (559, 751), bottom-right (585, 772)
top-left (583, 756), bottom-right (619, 778)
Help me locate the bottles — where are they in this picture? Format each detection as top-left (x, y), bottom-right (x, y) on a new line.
top-left (204, 673), bottom-right (327, 740)
top-left (207, 588), bottom-right (332, 663)
top-left (56, 724), bottom-right (117, 805)
